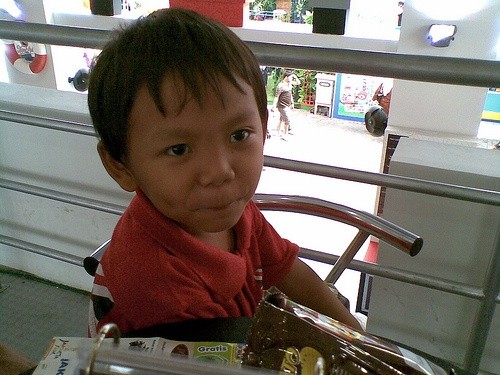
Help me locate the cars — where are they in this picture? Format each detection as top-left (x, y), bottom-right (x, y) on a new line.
top-left (255, 11), bottom-right (273, 20)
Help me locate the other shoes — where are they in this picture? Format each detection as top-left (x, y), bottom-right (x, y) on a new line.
top-left (288, 130), bottom-right (294, 134)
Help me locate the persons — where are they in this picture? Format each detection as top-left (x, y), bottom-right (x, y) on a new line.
top-left (271, 72), bottom-right (301, 137)
top-left (89, 8), bottom-right (368, 346)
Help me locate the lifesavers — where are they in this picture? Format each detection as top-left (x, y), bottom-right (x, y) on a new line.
top-left (4, 21), bottom-right (47, 74)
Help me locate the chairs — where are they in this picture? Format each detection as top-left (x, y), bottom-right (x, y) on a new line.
top-left (82, 193), bottom-right (425, 288)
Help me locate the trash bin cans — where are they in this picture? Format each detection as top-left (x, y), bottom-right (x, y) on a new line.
top-left (312, 73), bottom-right (335, 117)
